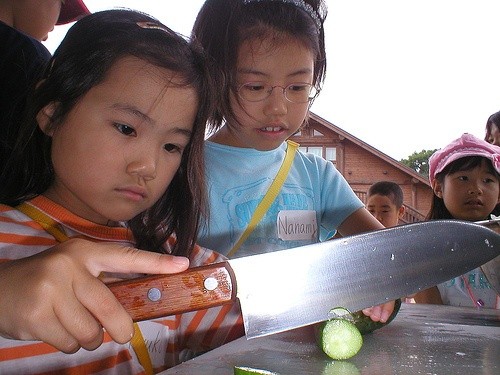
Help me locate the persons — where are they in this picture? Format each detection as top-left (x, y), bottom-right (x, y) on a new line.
top-left (364, 181), bottom-right (405, 228)
top-left (190, 0), bottom-right (396, 324)
top-left (0, 0), bottom-right (93, 207)
top-left (415, 110), bottom-right (500, 308)
top-left (0, 10), bottom-right (246, 375)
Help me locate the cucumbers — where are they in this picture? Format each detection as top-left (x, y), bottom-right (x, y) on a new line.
top-left (314, 298), bottom-right (403, 360)
top-left (234, 365), bottom-right (277, 375)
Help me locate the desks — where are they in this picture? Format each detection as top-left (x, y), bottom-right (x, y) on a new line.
top-left (154, 302), bottom-right (500, 375)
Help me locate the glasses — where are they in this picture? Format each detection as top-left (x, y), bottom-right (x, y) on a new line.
top-left (228, 74), bottom-right (321, 103)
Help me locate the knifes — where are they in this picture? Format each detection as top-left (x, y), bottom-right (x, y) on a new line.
top-left (97, 219), bottom-right (500, 342)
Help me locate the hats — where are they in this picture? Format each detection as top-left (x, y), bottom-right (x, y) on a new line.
top-left (428, 131), bottom-right (500, 190)
top-left (54, 0), bottom-right (92, 26)
